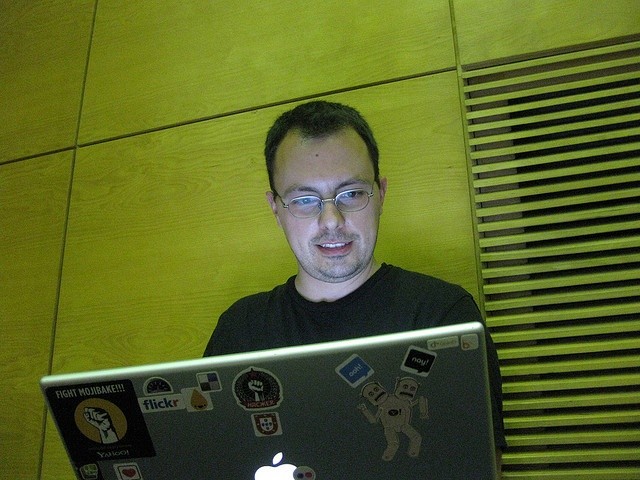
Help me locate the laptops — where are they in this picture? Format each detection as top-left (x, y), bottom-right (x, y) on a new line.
top-left (37, 320), bottom-right (500, 480)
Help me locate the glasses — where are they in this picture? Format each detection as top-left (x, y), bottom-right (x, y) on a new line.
top-left (272, 172), bottom-right (380, 219)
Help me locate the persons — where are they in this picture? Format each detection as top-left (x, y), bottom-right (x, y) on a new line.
top-left (202, 99), bottom-right (509, 480)
